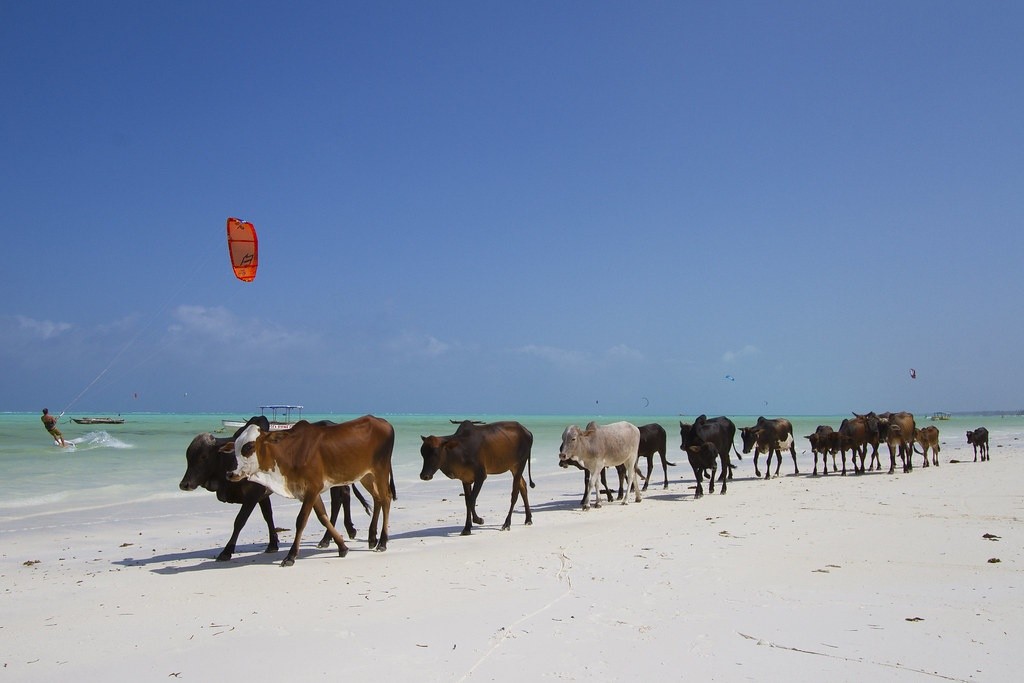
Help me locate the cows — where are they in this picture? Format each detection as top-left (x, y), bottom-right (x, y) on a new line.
top-left (738, 416), bottom-right (800, 480)
top-left (802, 411), bottom-right (940, 478)
top-left (966, 427), bottom-right (991, 462)
top-left (179, 413), bottom-right (398, 567)
top-left (559, 421), bottom-right (676, 510)
top-left (679, 415), bottom-right (742, 500)
top-left (419, 422), bottom-right (535, 537)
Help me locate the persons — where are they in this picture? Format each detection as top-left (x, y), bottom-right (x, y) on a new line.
top-left (41, 408), bottom-right (65, 447)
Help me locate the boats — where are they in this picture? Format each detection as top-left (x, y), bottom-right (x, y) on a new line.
top-left (222, 404), bottom-right (304, 432)
top-left (929, 411), bottom-right (952, 422)
top-left (69, 417), bottom-right (125, 424)
